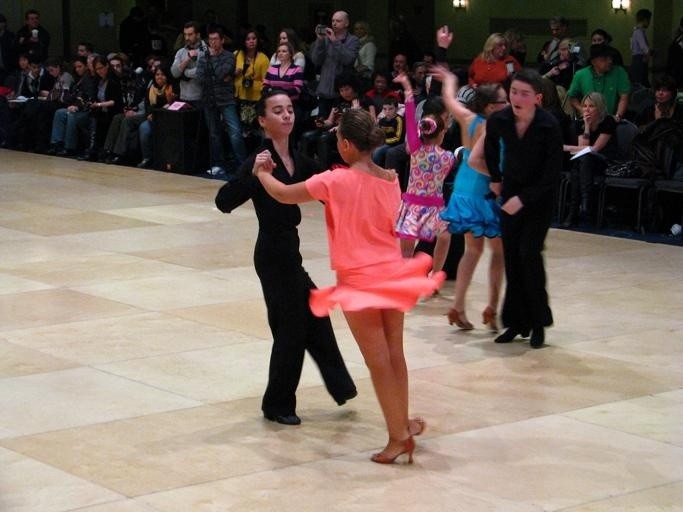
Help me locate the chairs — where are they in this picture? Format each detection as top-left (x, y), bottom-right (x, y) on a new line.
top-left (556, 171), bottom-right (683, 234)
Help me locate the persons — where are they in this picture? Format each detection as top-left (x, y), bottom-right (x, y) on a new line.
top-left (425, 63), bottom-right (512, 332)
top-left (394, 9), bottom-right (680, 299)
top-left (483, 68), bottom-right (566, 349)
top-left (0, 1), bottom-right (394, 178)
top-left (214, 87), bottom-right (399, 427)
top-left (253, 108), bottom-right (425, 463)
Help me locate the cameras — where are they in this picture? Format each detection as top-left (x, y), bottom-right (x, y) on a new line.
top-left (200, 45), bottom-right (209, 55)
top-left (242, 77), bottom-right (253, 88)
top-left (317, 25), bottom-right (327, 35)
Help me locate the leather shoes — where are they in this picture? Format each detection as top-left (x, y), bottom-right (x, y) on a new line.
top-left (264, 410), bottom-right (300, 425)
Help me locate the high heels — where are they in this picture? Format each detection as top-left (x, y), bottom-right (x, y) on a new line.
top-left (371, 436), bottom-right (415, 463)
top-left (408, 417), bottom-right (425, 435)
top-left (495, 325), bottom-right (529, 342)
top-left (448, 307), bottom-right (474, 330)
top-left (484, 307), bottom-right (499, 333)
top-left (530, 326), bottom-right (543, 348)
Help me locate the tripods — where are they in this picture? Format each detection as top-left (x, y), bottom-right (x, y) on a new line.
top-left (194, 60), bottom-right (232, 178)
